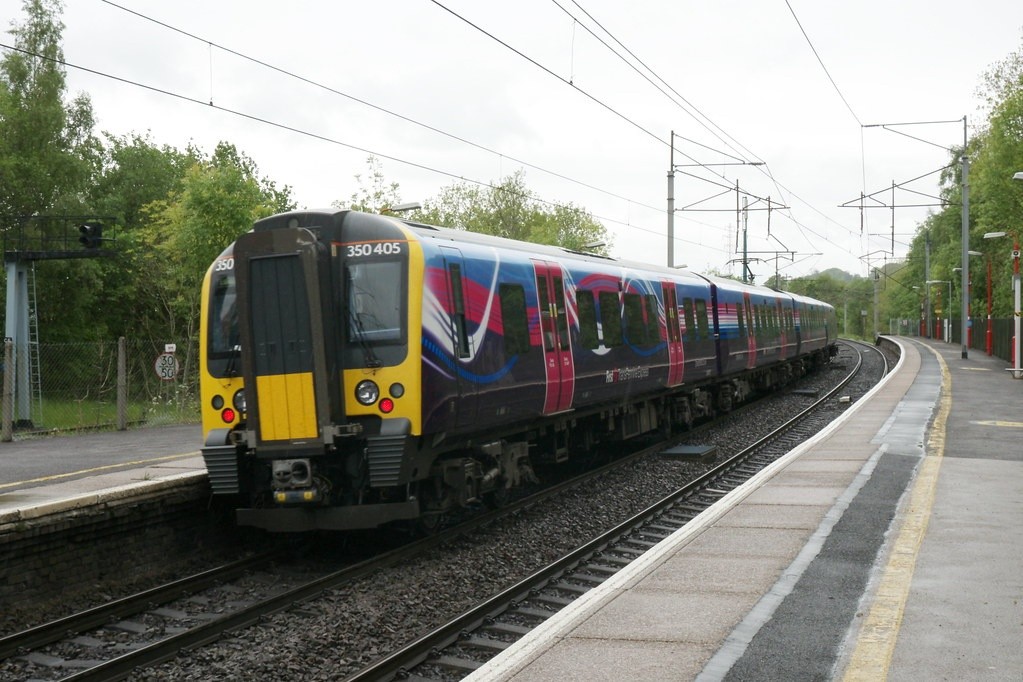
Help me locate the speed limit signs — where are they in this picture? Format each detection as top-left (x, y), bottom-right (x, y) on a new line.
top-left (154, 353), bottom-right (179, 380)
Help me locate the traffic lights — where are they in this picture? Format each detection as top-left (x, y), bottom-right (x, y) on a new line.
top-left (77, 222), bottom-right (102, 248)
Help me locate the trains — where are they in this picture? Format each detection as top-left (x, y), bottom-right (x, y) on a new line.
top-left (200, 208), bottom-right (837, 540)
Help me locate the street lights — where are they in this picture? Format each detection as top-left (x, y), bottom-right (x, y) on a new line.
top-left (967, 250), bottom-right (992, 357)
top-left (983, 229), bottom-right (1021, 368)
top-left (909, 279), bottom-right (943, 339)
top-left (952, 267), bottom-right (974, 348)
